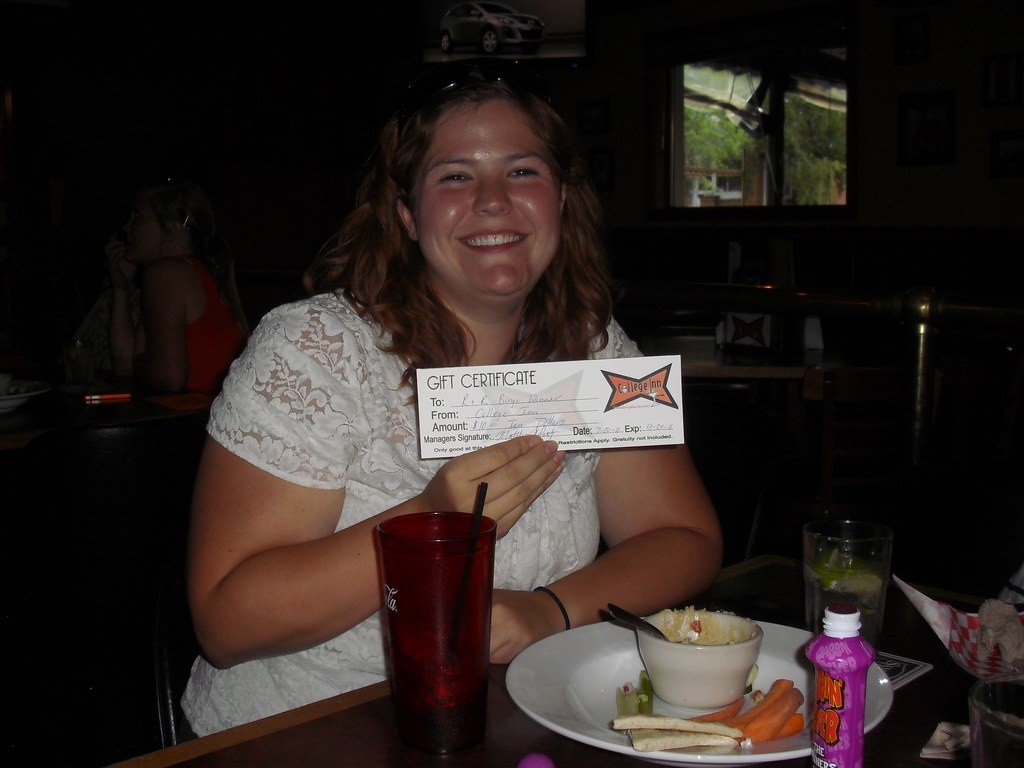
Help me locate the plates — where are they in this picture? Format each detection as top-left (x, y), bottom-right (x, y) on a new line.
top-left (0, 383), bottom-right (53, 414)
top-left (504, 619), bottom-right (895, 768)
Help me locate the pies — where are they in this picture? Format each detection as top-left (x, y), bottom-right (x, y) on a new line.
top-left (611, 712), bottom-right (743, 753)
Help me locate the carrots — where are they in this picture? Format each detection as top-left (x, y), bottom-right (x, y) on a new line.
top-left (684, 678), bottom-right (805, 744)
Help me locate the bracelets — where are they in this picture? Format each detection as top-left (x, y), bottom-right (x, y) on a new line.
top-left (533, 586), bottom-right (570, 630)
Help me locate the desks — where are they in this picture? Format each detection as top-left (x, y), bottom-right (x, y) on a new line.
top-left (109, 554), bottom-right (978, 768)
top-left (0, 395), bottom-right (216, 466)
top-left (645, 334), bottom-right (882, 560)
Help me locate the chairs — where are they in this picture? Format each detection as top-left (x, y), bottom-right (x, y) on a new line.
top-left (763, 363), bottom-right (913, 557)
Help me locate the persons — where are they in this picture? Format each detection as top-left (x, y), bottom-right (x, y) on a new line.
top-left (169, 71), bottom-right (725, 746)
top-left (105, 180), bottom-right (248, 413)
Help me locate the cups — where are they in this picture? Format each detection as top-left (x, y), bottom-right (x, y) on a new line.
top-left (803, 519), bottom-right (894, 646)
top-left (966, 672), bottom-right (1024, 768)
top-left (373, 511), bottom-right (498, 768)
top-left (62, 339), bottom-right (92, 393)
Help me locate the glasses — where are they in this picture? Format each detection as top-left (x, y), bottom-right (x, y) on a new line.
top-left (399, 56), bottom-right (546, 134)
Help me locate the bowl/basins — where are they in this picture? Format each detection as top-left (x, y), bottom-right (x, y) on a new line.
top-left (636, 609), bottom-right (764, 710)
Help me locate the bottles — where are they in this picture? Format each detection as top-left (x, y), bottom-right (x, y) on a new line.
top-left (803, 602), bottom-right (877, 768)
